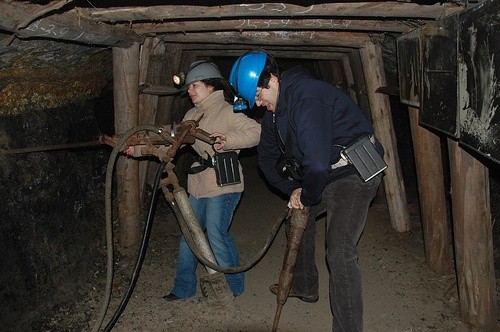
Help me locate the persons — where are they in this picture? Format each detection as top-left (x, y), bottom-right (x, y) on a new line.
top-left (228, 50), bottom-right (388, 331)
top-left (123, 61), bottom-right (265, 304)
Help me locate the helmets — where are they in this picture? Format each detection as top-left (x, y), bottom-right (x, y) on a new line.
top-left (228, 51), bottom-right (268, 111)
top-left (186, 60), bottom-right (224, 84)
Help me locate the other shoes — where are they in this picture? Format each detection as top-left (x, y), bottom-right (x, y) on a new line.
top-left (270, 283), bottom-right (320, 303)
top-left (161, 293), bottom-right (180, 301)
